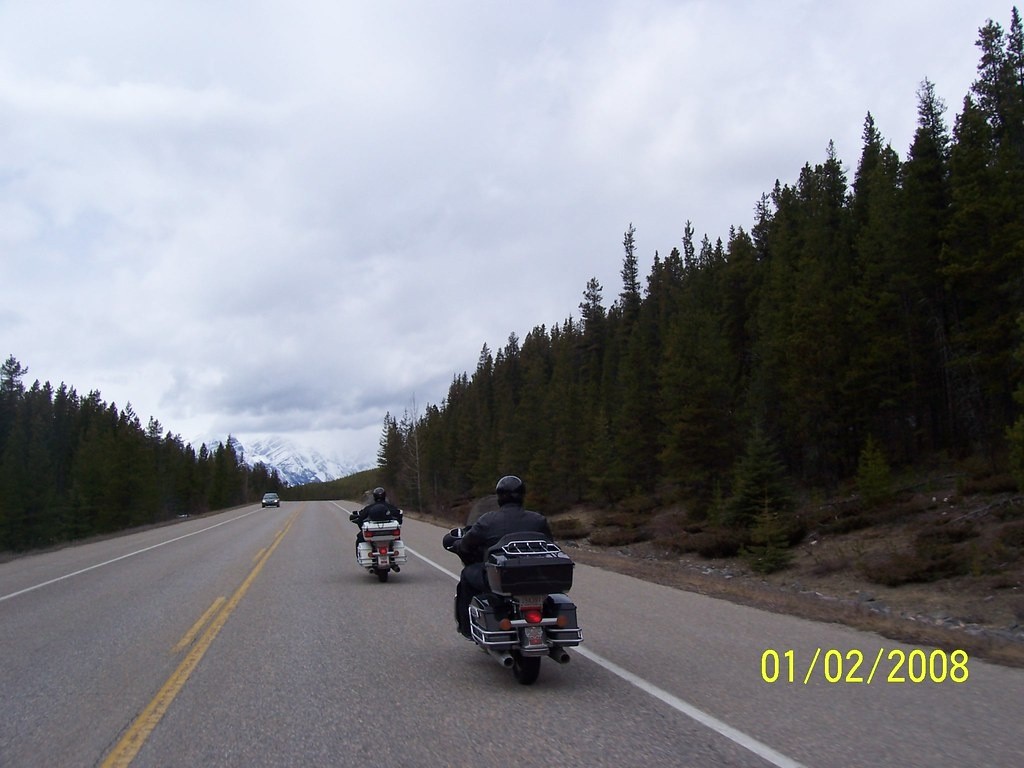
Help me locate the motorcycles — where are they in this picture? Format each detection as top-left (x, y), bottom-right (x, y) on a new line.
top-left (443, 526), bottom-right (585, 685)
top-left (349, 510), bottom-right (407, 582)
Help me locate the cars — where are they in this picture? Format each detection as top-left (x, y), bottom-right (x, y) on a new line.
top-left (261, 493), bottom-right (280, 508)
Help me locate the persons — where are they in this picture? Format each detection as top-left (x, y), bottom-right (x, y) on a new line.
top-left (449, 476), bottom-right (554, 641)
top-left (350, 487), bottom-right (404, 559)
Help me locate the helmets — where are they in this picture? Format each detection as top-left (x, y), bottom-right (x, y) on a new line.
top-left (496, 475), bottom-right (524, 508)
top-left (373, 487), bottom-right (385, 502)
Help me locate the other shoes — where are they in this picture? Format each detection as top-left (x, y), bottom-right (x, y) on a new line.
top-left (390, 562), bottom-right (400, 572)
top-left (456, 627), bottom-right (473, 641)
